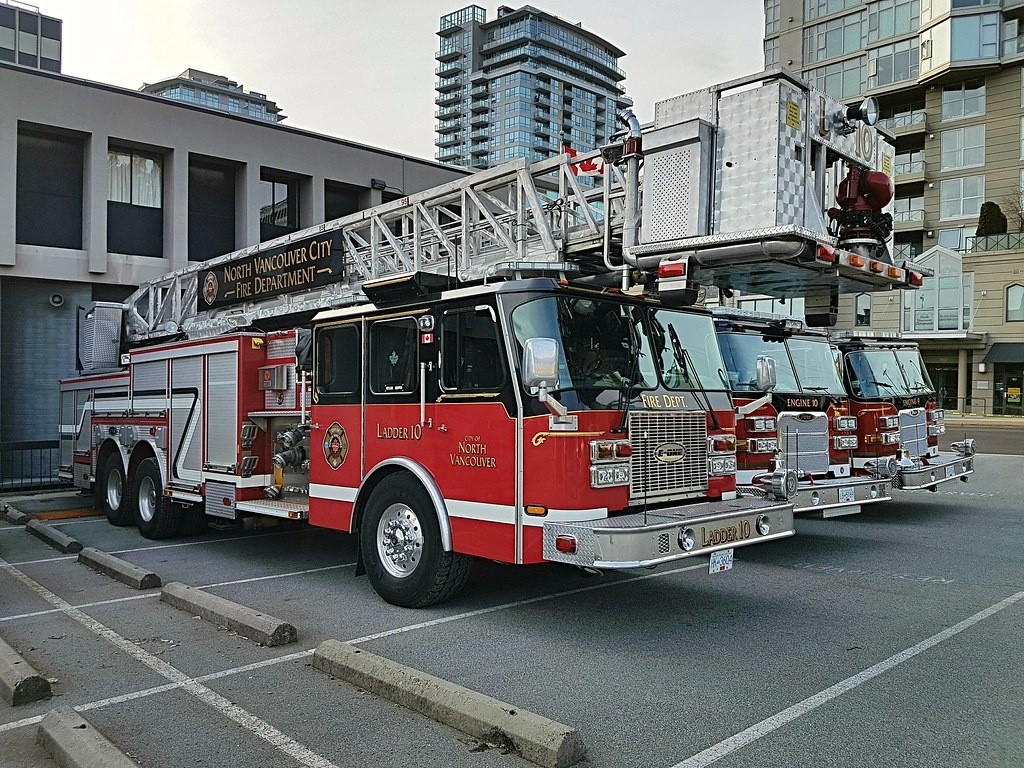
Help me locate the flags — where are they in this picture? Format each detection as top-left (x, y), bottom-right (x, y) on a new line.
top-left (562, 146), bottom-right (605, 177)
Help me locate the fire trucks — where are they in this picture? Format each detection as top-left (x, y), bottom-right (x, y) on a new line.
top-left (829, 331), bottom-right (977, 491)
top-left (52, 66), bottom-right (935, 608)
top-left (698, 305), bottom-right (898, 520)
